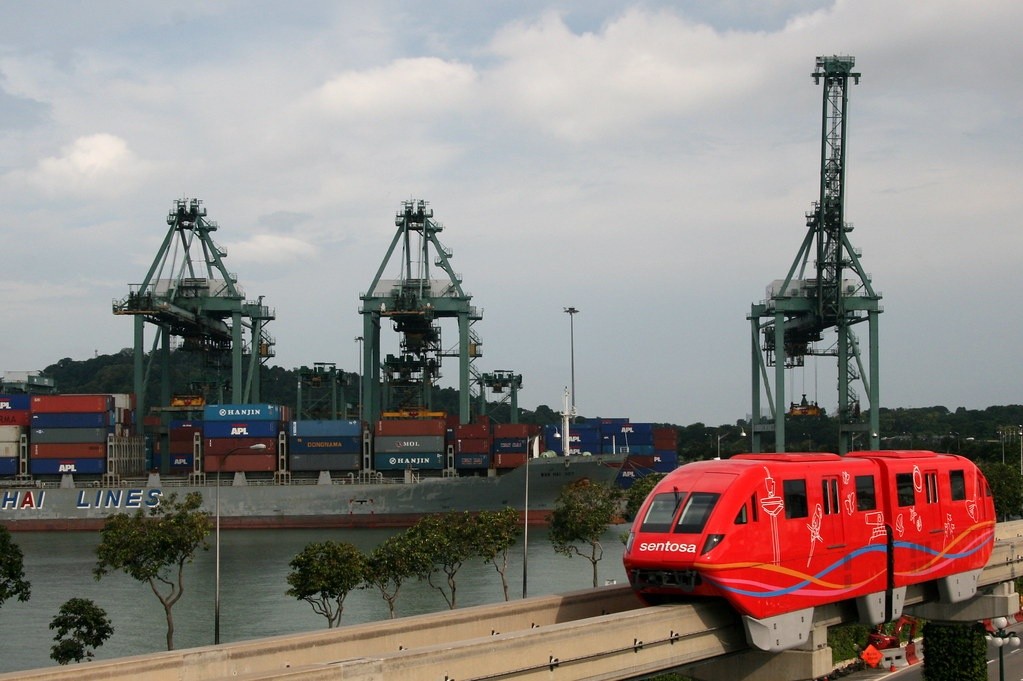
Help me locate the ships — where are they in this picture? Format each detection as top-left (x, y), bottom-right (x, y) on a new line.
top-left (0, 395), bottom-right (626, 532)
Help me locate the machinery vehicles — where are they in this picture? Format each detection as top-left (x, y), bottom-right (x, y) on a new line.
top-left (853, 615), bottom-right (919, 666)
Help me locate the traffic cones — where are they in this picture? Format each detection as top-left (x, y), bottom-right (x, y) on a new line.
top-left (888, 657), bottom-right (896, 672)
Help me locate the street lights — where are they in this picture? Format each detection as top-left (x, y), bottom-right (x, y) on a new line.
top-left (718, 424), bottom-right (746, 458)
top-left (562, 307), bottom-right (579, 423)
top-left (904, 430), bottom-right (912, 449)
top-left (852, 429), bottom-right (878, 451)
top-left (213, 444), bottom-right (264, 643)
top-left (522, 424), bottom-right (563, 597)
top-left (804, 433), bottom-right (811, 451)
top-left (947, 434), bottom-right (974, 454)
top-left (949, 432), bottom-right (960, 453)
top-left (1001, 425), bottom-right (1022, 463)
top-left (354, 337), bottom-right (364, 419)
top-left (985, 618), bottom-right (1021, 681)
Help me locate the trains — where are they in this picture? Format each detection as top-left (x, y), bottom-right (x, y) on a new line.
top-left (623, 450), bottom-right (996, 654)
